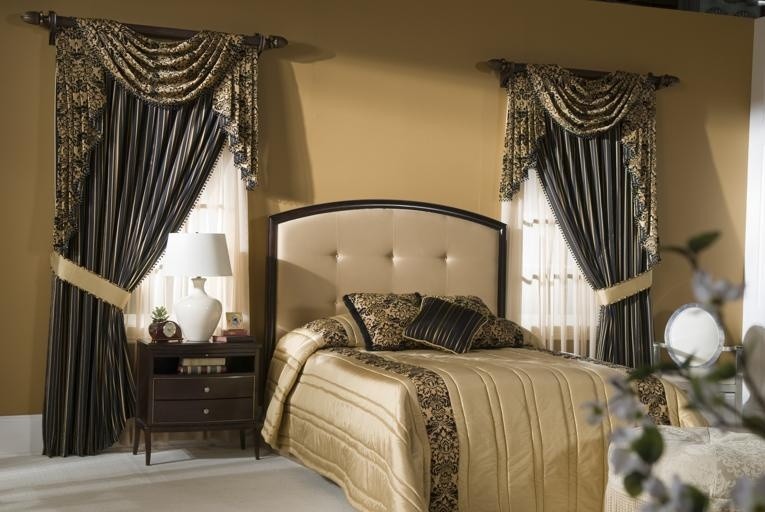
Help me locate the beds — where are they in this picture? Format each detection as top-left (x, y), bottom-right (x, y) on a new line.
top-left (265, 199), bottom-right (697, 509)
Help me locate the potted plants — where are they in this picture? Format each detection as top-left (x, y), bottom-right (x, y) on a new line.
top-left (148, 305), bottom-right (170, 342)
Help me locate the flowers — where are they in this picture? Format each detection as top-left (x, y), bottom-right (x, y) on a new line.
top-left (582, 227), bottom-right (763, 509)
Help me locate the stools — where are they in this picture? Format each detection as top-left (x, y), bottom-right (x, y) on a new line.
top-left (604, 423), bottom-right (764, 510)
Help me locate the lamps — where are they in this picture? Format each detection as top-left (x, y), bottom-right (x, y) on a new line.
top-left (164, 231), bottom-right (235, 343)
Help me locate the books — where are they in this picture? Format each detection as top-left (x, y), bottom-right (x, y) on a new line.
top-left (178, 358), bottom-right (226, 375)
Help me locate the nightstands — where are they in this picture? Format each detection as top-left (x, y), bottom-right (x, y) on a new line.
top-left (132, 334), bottom-right (264, 467)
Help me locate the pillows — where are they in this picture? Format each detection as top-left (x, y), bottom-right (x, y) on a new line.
top-left (341, 288), bottom-right (527, 354)
